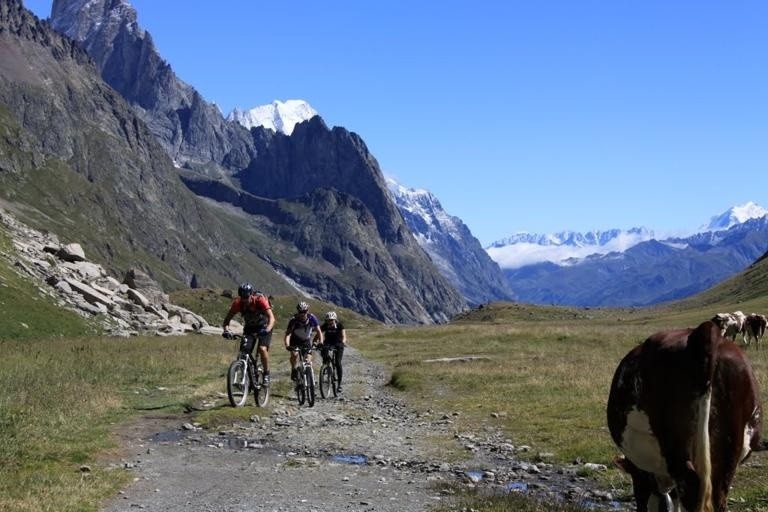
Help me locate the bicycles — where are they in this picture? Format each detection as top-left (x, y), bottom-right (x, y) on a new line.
top-left (311, 343), bottom-right (344, 402)
top-left (285, 342), bottom-right (324, 409)
top-left (220, 330), bottom-right (275, 412)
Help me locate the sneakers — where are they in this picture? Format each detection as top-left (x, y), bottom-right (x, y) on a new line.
top-left (290, 368), bottom-right (298, 381)
top-left (261, 374), bottom-right (271, 388)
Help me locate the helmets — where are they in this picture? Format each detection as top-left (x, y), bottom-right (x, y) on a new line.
top-left (296, 302), bottom-right (311, 311)
top-left (237, 284), bottom-right (253, 297)
top-left (324, 311), bottom-right (337, 320)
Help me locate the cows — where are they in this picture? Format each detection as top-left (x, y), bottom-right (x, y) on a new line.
top-left (605, 319), bottom-right (762, 512)
top-left (711, 309), bottom-right (768, 351)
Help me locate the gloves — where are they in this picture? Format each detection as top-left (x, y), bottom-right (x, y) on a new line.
top-left (317, 343), bottom-right (325, 351)
top-left (259, 328), bottom-right (269, 337)
top-left (287, 345), bottom-right (298, 352)
top-left (335, 342), bottom-right (345, 350)
top-left (221, 330), bottom-right (233, 339)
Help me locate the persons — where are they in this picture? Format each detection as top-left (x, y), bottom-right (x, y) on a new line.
top-left (284, 301), bottom-right (325, 395)
top-left (311, 310), bottom-right (347, 392)
top-left (222, 282), bottom-right (276, 394)
